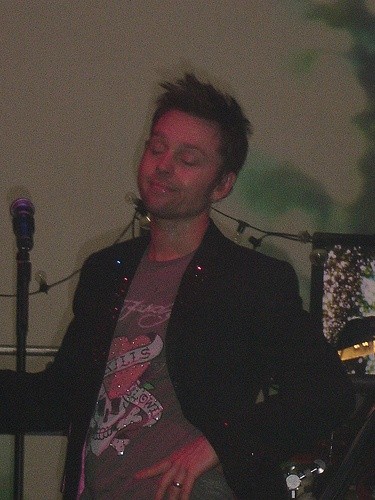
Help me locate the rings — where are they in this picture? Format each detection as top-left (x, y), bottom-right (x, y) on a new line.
top-left (172, 481), bottom-right (183, 488)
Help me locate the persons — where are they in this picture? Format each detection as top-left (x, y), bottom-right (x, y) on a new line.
top-left (0, 74), bottom-right (356, 500)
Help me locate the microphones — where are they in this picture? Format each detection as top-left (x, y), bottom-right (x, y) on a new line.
top-left (9, 197), bottom-right (35, 252)
